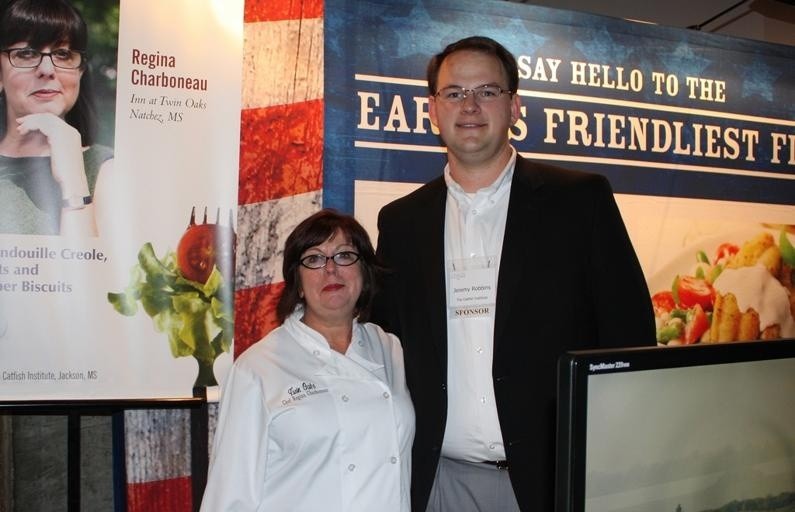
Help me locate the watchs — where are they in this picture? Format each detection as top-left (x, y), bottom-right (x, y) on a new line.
top-left (62, 196), bottom-right (92, 209)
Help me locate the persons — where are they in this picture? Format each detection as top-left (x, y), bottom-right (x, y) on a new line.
top-left (375, 36), bottom-right (657, 512)
top-left (0, 0), bottom-right (115, 238)
top-left (199, 206), bottom-right (416, 512)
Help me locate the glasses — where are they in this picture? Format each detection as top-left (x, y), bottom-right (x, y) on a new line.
top-left (434, 83), bottom-right (512, 104)
top-left (294, 252), bottom-right (363, 269)
top-left (2, 47), bottom-right (87, 69)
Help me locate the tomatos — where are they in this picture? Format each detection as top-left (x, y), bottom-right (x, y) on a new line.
top-left (177, 225), bottom-right (237, 285)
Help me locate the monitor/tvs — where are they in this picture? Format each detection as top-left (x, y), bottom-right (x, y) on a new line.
top-left (552, 337), bottom-right (795, 512)
